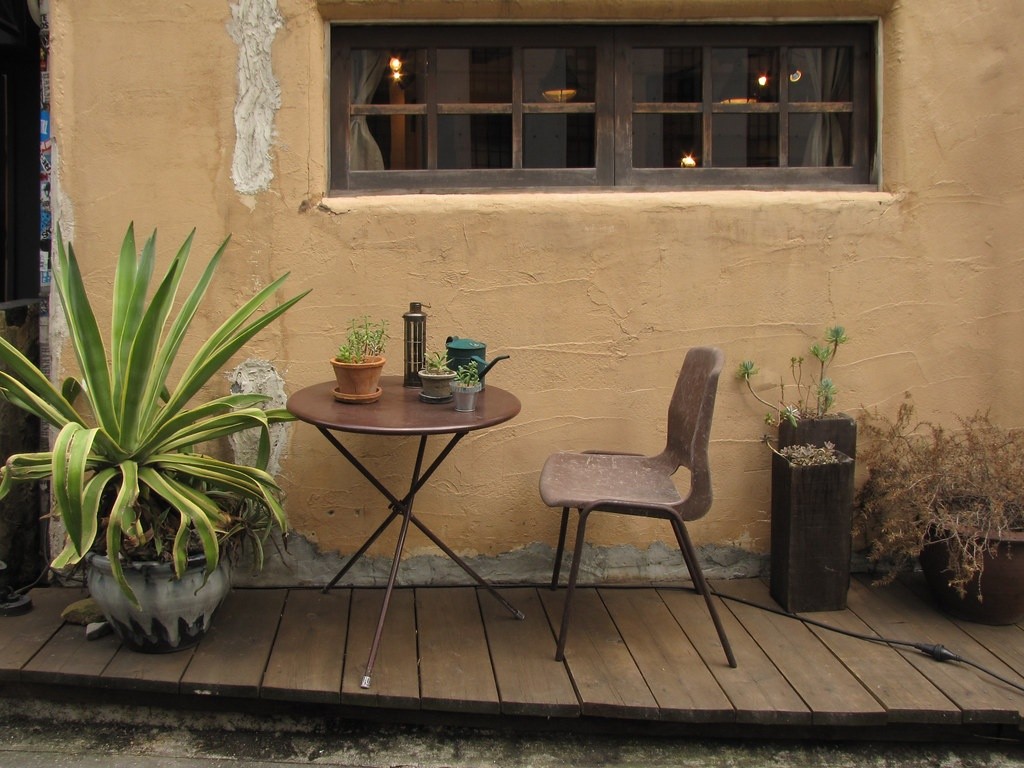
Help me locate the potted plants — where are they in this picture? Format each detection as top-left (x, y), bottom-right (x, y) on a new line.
top-left (734, 326), bottom-right (857, 459)
top-left (449, 361), bottom-right (482, 413)
top-left (852, 391), bottom-right (1024, 627)
top-left (0, 215), bottom-right (315, 653)
top-left (417, 350), bottom-right (457, 404)
top-left (761, 432), bottom-right (854, 613)
top-left (331, 314), bottom-right (391, 405)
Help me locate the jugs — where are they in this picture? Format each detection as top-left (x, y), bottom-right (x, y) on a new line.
top-left (446, 335), bottom-right (510, 391)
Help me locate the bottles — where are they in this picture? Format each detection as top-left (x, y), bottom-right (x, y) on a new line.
top-left (401, 302), bottom-right (427, 389)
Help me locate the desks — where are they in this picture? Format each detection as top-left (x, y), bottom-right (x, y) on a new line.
top-left (286, 376), bottom-right (526, 688)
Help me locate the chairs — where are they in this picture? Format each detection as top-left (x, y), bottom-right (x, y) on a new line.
top-left (538, 342), bottom-right (738, 670)
